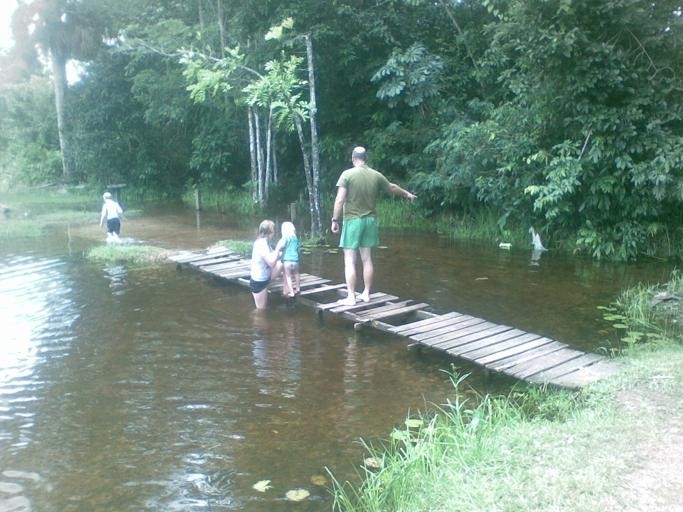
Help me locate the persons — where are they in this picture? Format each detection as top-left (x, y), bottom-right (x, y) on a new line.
top-left (331, 146), bottom-right (417, 305)
top-left (250, 220), bottom-right (290, 309)
top-left (100, 192), bottom-right (126, 241)
top-left (281, 222), bottom-right (301, 297)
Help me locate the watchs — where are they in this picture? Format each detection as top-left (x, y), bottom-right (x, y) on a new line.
top-left (332, 218), bottom-right (338, 222)
